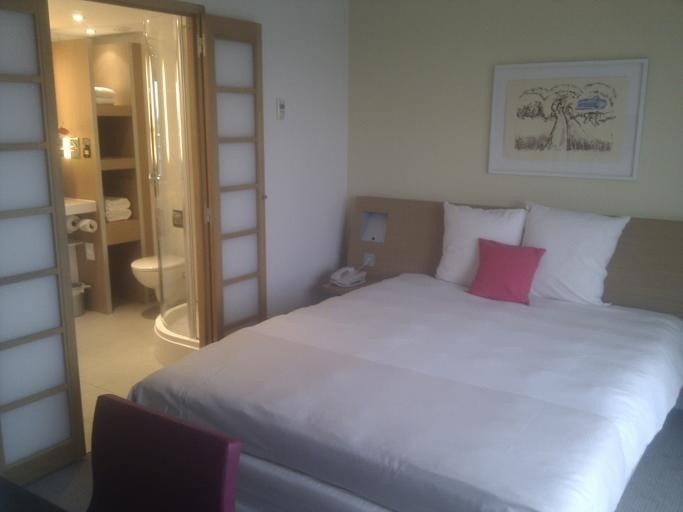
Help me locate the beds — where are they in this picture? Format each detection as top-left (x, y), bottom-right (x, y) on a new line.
top-left (126, 194), bottom-right (681, 512)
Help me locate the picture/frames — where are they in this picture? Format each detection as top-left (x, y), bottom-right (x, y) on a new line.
top-left (485, 58), bottom-right (649, 183)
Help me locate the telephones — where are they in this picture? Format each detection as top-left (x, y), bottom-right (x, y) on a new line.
top-left (329, 265), bottom-right (367, 287)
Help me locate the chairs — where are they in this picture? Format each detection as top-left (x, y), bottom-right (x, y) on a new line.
top-left (85, 391), bottom-right (242, 510)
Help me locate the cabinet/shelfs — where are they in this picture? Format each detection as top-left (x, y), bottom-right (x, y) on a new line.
top-left (51, 41), bottom-right (156, 314)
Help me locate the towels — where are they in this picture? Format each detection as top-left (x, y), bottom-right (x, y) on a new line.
top-left (92, 86), bottom-right (116, 106)
top-left (105, 196), bottom-right (132, 222)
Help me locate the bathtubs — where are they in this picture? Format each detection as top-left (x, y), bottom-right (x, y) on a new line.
top-left (152, 299), bottom-right (199, 362)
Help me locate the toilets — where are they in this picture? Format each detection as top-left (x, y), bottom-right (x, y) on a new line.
top-left (131, 192), bottom-right (186, 307)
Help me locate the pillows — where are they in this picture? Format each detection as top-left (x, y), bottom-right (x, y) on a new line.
top-left (435, 200), bottom-right (632, 308)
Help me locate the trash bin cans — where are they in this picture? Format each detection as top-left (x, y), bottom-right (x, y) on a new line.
top-left (72, 281), bottom-right (87, 318)
